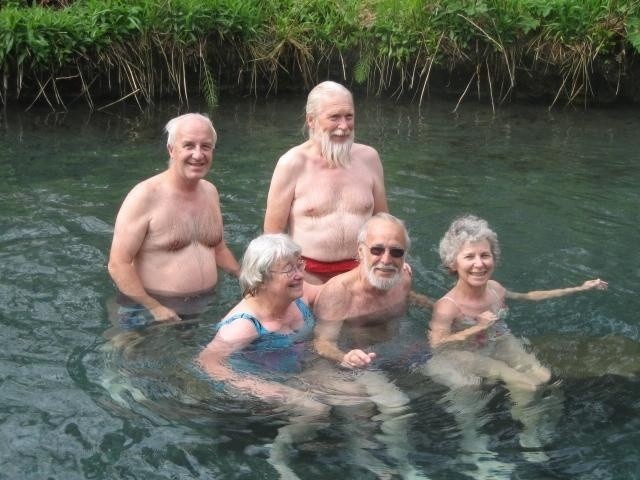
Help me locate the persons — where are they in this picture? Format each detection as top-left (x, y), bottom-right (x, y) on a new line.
top-left (427, 213), bottom-right (609, 466)
top-left (312, 210), bottom-right (514, 479)
top-left (106, 113), bottom-right (242, 341)
top-left (262, 78), bottom-right (390, 306)
top-left (194, 232), bottom-right (412, 480)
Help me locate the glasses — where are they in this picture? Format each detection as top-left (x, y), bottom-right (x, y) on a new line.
top-left (267, 260), bottom-right (305, 278)
top-left (362, 242), bottom-right (405, 258)
